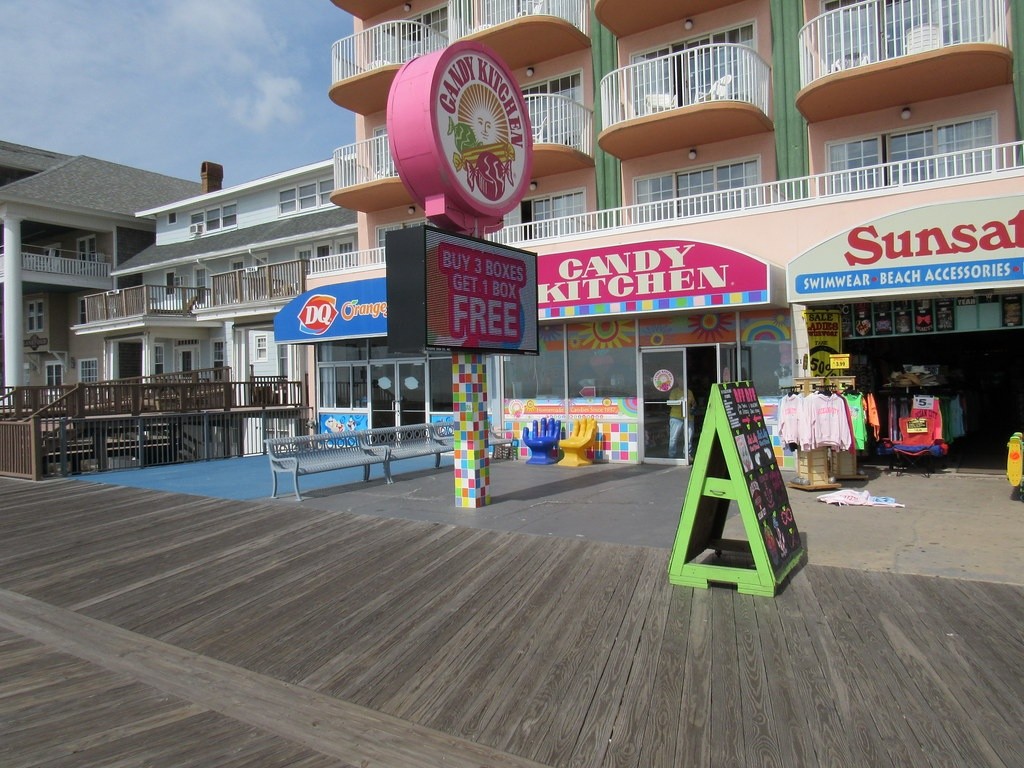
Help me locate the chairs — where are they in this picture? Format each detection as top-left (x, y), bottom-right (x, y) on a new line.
top-left (646, 94), bottom-right (677, 114)
top-left (906, 22), bottom-right (939, 55)
top-left (531, 116), bottom-right (547, 142)
top-left (523, 418), bottom-right (561, 465)
top-left (474, 24), bottom-right (493, 34)
top-left (521, 4), bottom-right (543, 16)
top-left (557, 416), bottom-right (597, 466)
top-left (148, 296), bottom-right (198, 314)
top-left (697, 75), bottom-right (732, 101)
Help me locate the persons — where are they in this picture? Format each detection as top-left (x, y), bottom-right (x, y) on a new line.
top-left (667, 372), bottom-right (697, 465)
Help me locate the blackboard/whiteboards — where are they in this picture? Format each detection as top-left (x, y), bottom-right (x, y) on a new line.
top-left (665, 378), bottom-right (808, 598)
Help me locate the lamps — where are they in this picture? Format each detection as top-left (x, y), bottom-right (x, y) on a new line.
top-left (404, 3), bottom-right (411, 12)
top-left (688, 149), bottom-right (696, 160)
top-left (408, 206), bottom-right (416, 215)
top-left (526, 67), bottom-right (534, 77)
top-left (530, 181), bottom-right (538, 190)
top-left (684, 19), bottom-right (693, 30)
top-left (900, 106), bottom-right (911, 120)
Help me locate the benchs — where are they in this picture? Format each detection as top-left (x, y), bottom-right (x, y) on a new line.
top-left (263, 430), bottom-right (391, 502)
top-left (361, 423), bottom-right (454, 484)
top-left (432, 422), bottom-right (512, 469)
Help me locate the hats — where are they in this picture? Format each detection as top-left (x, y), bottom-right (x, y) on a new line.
top-left (894, 372), bottom-right (921, 388)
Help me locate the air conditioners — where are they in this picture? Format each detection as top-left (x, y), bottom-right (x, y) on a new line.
top-left (190, 223), bottom-right (204, 234)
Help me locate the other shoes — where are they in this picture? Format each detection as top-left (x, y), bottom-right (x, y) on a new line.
top-left (683, 454), bottom-right (694, 464)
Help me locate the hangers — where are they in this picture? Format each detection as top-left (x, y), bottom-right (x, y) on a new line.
top-left (819, 385), bottom-right (835, 396)
top-left (845, 384), bottom-right (873, 394)
top-left (789, 386), bottom-right (799, 397)
